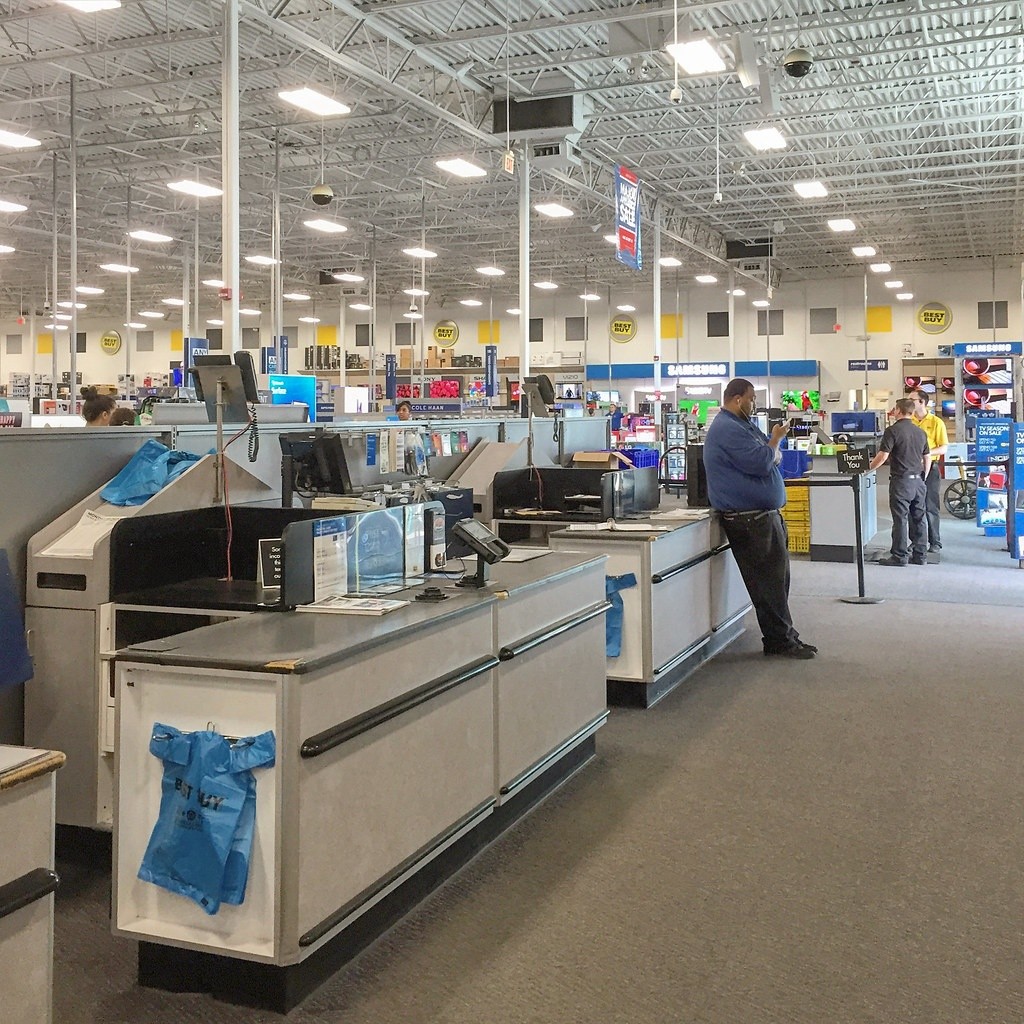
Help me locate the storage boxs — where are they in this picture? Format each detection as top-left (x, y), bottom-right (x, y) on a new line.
top-left (12, 373), bottom-right (83, 400)
top-left (399, 345), bottom-right (563, 369)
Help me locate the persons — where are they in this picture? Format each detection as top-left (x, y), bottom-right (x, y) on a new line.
top-left (703, 379), bottom-right (818, 659)
top-left (109, 408), bottom-right (139, 426)
top-left (80, 386), bottom-right (117, 426)
top-left (587, 392), bottom-right (601, 401)
top-left (801, 391), bottom-right (812, 410)
top-left (907, 390), bottom-right (948, 553)
top-left (787, 399), bottom-right (800, 410)
top-left (565, 388), bottom-right (574, 398)
top-left (396, 401), bottom-right (412, 421)
top-left (870, 398), bottom-right (932, 566)
top-left (691, 402), bottom-right (700, 423)
top-left (605, 403), bottom-right (628, 431)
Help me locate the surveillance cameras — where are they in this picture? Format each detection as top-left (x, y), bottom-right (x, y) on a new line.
top-left (714, 193), bottom-right (723, 203)
top-left (784, 49), bottom-right (813, 78)
top-left (670, 87), bottom-right (682, 105)
top-left (310, 186), bottom-right (333, 206)
top-left (43, 302), bottom-right (51, 309)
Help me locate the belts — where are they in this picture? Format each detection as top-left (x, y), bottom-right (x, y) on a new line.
top-left (721, 508), bottom-right (780, 521)
top-left (892, 475), bottom-right (921, 479)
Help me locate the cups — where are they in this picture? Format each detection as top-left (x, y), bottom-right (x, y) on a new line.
top-left (942, 378), bottom-right (954, 394)
top-left (905, 377), bottom-right (922, 393)
top-left (964, 389), bottom-right (991, 413)
top-left (962, 359), bottom-right (990, 379)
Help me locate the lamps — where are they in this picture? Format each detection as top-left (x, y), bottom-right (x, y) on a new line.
top-left (0, 0), bottom-right (913, 323)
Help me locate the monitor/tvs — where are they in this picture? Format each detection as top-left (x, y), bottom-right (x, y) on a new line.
top-left (278, 432), bottom-right (354, 496)
top-left (810, 425), bottom-right (833, 445)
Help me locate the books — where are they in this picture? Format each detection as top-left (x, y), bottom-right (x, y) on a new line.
top-left (650, 512), bottom-right (709, 520)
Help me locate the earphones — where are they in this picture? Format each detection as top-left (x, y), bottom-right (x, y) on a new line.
top-left (737, 399), bottom-right (740, 403)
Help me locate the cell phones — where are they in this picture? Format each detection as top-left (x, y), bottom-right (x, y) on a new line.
top-left (782, 418), bottom-right (793, 427)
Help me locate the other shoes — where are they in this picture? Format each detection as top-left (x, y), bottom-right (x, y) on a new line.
top-left (929, 545), bottom-right (940, 553)
top-left (908, 556), bottom-right (924, 565)
top-left (761, 636), bottom-right (815, 659)
top-left (788, 630), bottom-right (818, 652)
top-left (884, 555), bottom-right (907, 566)
top-left (907, 543), bottom-right (915, 552)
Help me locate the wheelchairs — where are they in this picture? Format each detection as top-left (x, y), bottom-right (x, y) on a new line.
top-left (944, 456), bottom-right (976, 520)
